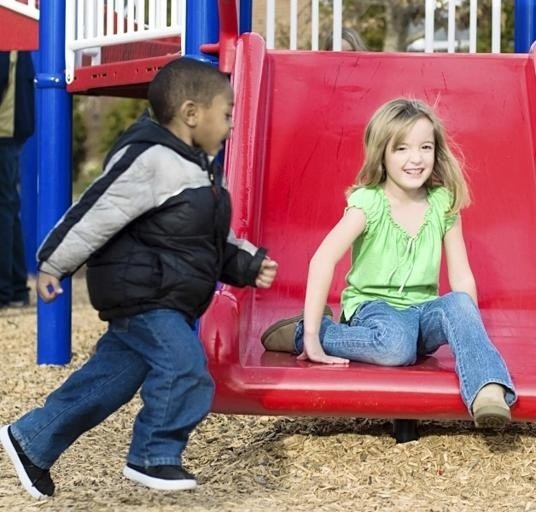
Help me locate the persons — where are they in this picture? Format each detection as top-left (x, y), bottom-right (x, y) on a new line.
top-left (0, 56), bottom-right (279, 501)
top-left (324, 27), bottom-right (369, 52)
top-left (260, 97), bottom-right (519, 430)
top-left (0, 51), bottom-right (36, 311)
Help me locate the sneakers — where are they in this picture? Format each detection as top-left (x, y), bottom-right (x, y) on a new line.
top-left (122, 462), bottom-right (196, 491)
top-left (0, 424), bottom-right (54, 499)
top-left (261, 306), bottom-right (332, 354)
top-left (473, 387), bottom-right (511, 428)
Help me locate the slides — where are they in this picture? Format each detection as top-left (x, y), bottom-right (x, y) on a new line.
top-left (195, 31), bottom-right (536, 421)
